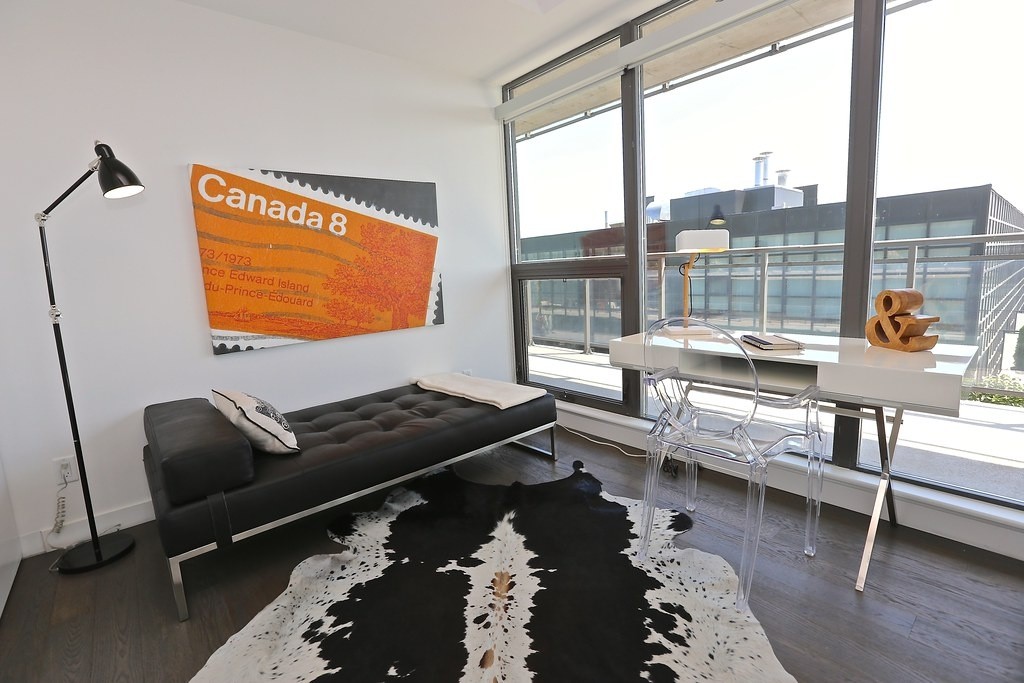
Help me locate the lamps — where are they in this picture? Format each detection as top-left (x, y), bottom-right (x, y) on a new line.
top-left (660, 229), bottom-right (729, 334)
top-left (34, 139), bottom-right (144, 578)
top-left (703, 204), bottom-right (726, 325)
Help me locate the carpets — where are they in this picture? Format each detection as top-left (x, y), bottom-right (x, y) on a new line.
top-left (188, 461), bottom-right (799, 683)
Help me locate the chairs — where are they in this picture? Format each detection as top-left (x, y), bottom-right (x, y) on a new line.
top-left (635, 317), bottom-right (827, 613)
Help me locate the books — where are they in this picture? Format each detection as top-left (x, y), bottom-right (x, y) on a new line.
top-left (740, 333), bottom-right (806, 350)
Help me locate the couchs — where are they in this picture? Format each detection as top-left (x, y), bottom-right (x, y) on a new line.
top-left (140, 370), bottom-right (558, 623)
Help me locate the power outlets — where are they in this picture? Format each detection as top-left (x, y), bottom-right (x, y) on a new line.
top-left (51, 455), bottom-right (79, 486)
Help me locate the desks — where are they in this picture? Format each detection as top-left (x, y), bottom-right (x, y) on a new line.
top-left (609, 325), bottom-right (978, 593)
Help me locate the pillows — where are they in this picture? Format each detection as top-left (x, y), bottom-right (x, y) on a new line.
top-left (143, 398), bottom-right (255, 507)
top-left (211, 385), bottom-right (301, 457)
